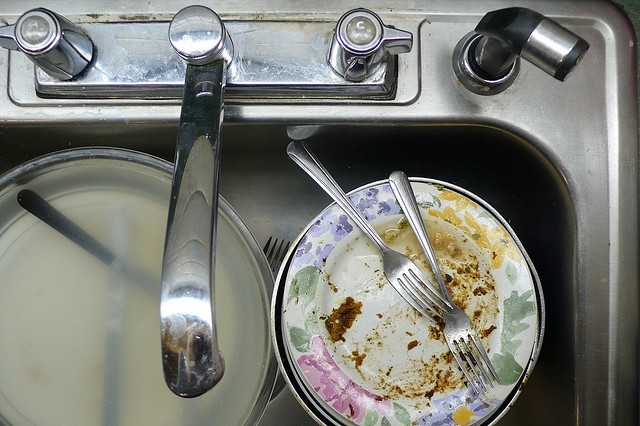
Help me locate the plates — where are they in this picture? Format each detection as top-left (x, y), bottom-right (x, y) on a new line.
top-left (270, 177), bottom-right (546, 426)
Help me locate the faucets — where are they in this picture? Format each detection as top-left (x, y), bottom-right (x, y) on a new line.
top-left (160, 4), bottom-right (235, 399)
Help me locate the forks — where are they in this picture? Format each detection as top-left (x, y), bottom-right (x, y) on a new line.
top-left (286, 141), bottom-right (454, 324)
top-left (388, 170), bottom-right (501, 395)
top-left (263, 237), bottom-right (291, 273)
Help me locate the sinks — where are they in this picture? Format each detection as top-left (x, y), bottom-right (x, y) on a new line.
top-left (0, 117), bottom-right (583, 426)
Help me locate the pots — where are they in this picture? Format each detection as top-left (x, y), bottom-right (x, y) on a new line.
top-left (1, 145), bottom-right (279, 426)
